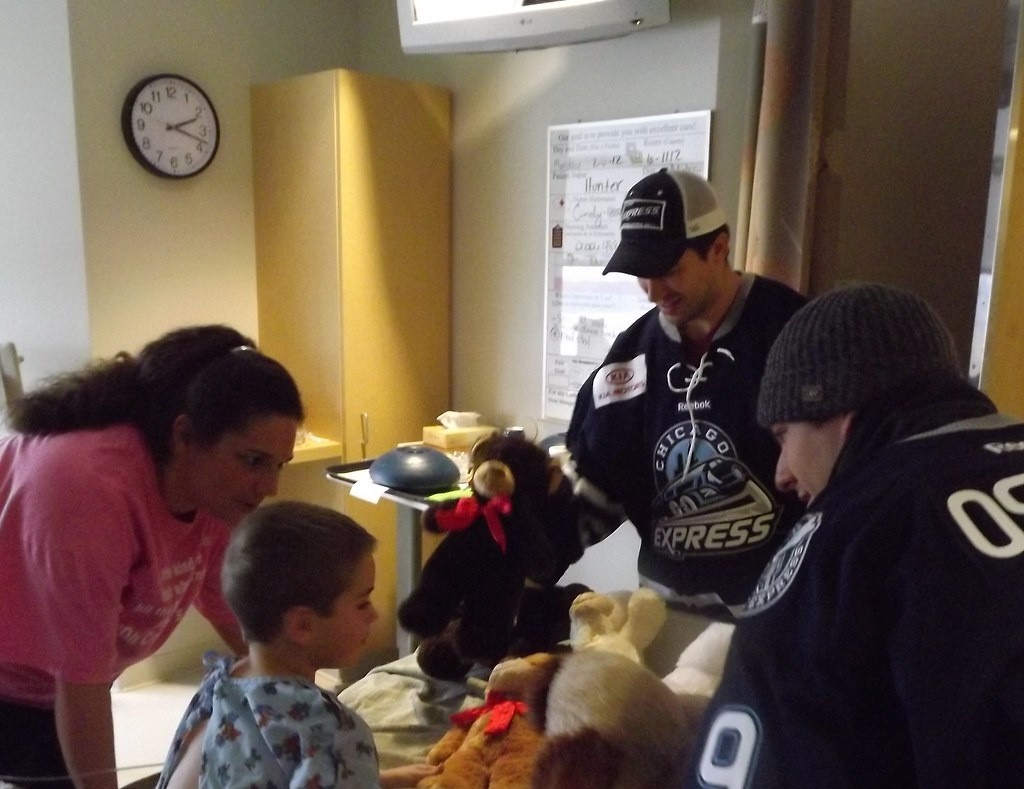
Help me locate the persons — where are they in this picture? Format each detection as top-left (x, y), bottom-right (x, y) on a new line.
top-left (0, 324), bottom-right (307, 789)
top-left (153, 497), bottom-right (381, 788)
top-left (690, 281), bottom-right (1023, 788)
top-left (564, 168), bottom-right (822, 608)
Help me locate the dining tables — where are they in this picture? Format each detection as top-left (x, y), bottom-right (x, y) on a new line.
top-left (326, 432), bottom-right (554, 658)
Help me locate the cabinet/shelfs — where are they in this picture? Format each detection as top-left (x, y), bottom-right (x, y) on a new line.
top-left (248, 67), bottom-right (453, 698)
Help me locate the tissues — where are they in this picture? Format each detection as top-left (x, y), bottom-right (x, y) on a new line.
top-left (422, 410), bottom-right (501, 454)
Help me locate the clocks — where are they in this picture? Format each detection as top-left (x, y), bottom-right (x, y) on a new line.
top-left (121, 72), bottom-right (221, 180)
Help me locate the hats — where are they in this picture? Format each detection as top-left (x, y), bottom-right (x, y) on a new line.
top-left (757, 283), bottom-right (960, 426)
top-left (602, 167), bottom-right (726, 277)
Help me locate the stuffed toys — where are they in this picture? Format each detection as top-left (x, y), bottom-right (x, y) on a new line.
top-left (416, 588), bottom-right (711, 788)
top-left (394, 428), bottom-right (593, 679)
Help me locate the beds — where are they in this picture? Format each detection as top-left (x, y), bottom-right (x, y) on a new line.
top-left (120, 588), bottom-right (737, 789)
